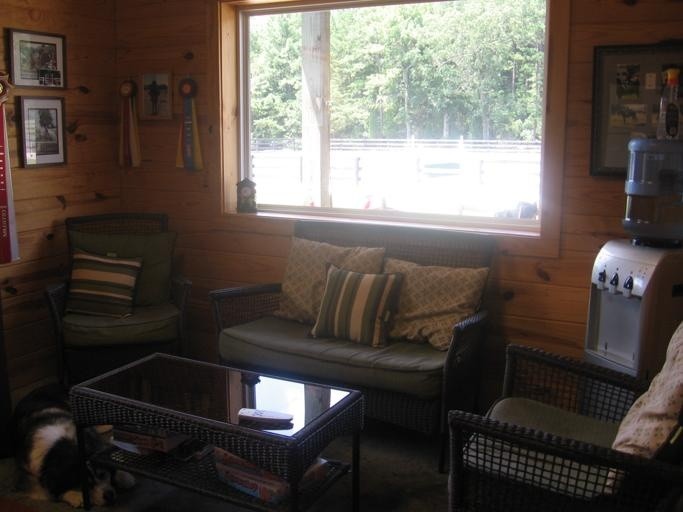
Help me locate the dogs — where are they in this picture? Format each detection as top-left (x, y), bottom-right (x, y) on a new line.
top-left (11, 406), bottom-right (135, 507)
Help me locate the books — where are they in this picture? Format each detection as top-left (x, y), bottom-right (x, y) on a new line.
top-left (111, 424), bottom-right (193, 452)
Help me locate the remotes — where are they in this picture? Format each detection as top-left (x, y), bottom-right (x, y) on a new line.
top-left (237, 408), bottom-right (293, 423)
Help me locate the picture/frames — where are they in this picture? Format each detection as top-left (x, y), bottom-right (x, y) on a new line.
top-left (138, 71), bottom-right (173, 121)
top-left (9, 28), bottom-right (67, 89)
top-left (17, 96), bottom-right (67, 168)
top-left (589, 43), bottom-right (683, 177)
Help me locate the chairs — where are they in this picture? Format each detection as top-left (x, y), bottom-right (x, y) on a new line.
top-left (447, 320), bottom-right (683, 512)
top-left (44, 212), bottom-right (192, 382)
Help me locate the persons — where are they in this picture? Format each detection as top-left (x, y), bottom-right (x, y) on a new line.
top-left (149, 80), bottom-right (161, 113)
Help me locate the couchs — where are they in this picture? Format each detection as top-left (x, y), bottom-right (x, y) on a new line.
top-left (208, 221), bottom-right (504, 472)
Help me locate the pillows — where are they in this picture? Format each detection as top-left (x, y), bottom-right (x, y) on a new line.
top-left (64, 247), bottom-right (143, 318)
top-left (382, 258), bottom-right (489, 353)
top-left (307, 263), bottom-right (405, 347)
top-left (272, 236), bottom-right (386, 324)
top-left (603, 322), bottom-right (683, 494)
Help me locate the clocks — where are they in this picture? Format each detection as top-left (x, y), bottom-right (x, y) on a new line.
top-left (236, 177), bottom-right (257, 213)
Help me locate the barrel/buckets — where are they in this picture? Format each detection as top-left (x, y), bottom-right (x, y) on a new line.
top-left (621, 138), bottom-right (683, 246)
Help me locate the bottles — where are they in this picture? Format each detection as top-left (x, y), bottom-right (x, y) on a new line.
top-left (656, 68), bottom-right (681, 141)
